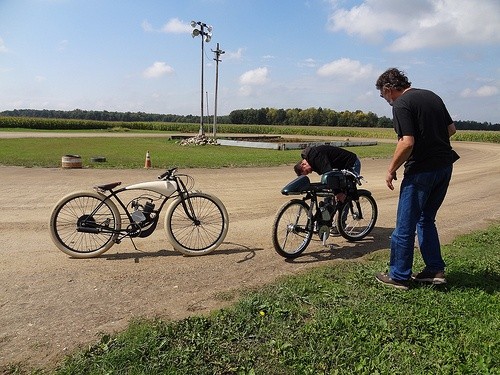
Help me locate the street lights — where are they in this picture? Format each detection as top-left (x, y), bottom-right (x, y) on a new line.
top-left (190, 21), bottom-right (214, 137)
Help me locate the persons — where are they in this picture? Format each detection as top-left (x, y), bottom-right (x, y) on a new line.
top-left (293, 145), bottom-right (361, 237)
top-left (375, 67), bottom-right (459, 291)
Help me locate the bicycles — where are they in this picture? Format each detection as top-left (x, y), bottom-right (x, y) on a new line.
top-left (48, 167), bottom-right (230, 260)
top-left (272, 167), bottom-right (378, 259)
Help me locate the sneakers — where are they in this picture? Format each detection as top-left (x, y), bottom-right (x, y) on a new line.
top-left (313, 225), bottom-right (319, 233)
top-left (330, 224), bottom-right (348, 236)
top-left (375, 272), bottom-right (408, 288)
top-left (411, 268), bottom-right (446, 284)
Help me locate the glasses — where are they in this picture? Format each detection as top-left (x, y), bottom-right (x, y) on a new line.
top-left (379, 83), bottom-right (390, 98)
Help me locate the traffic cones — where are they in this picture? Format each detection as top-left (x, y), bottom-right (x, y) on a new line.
top-left (143, 150), bottom-right (152, 168)
